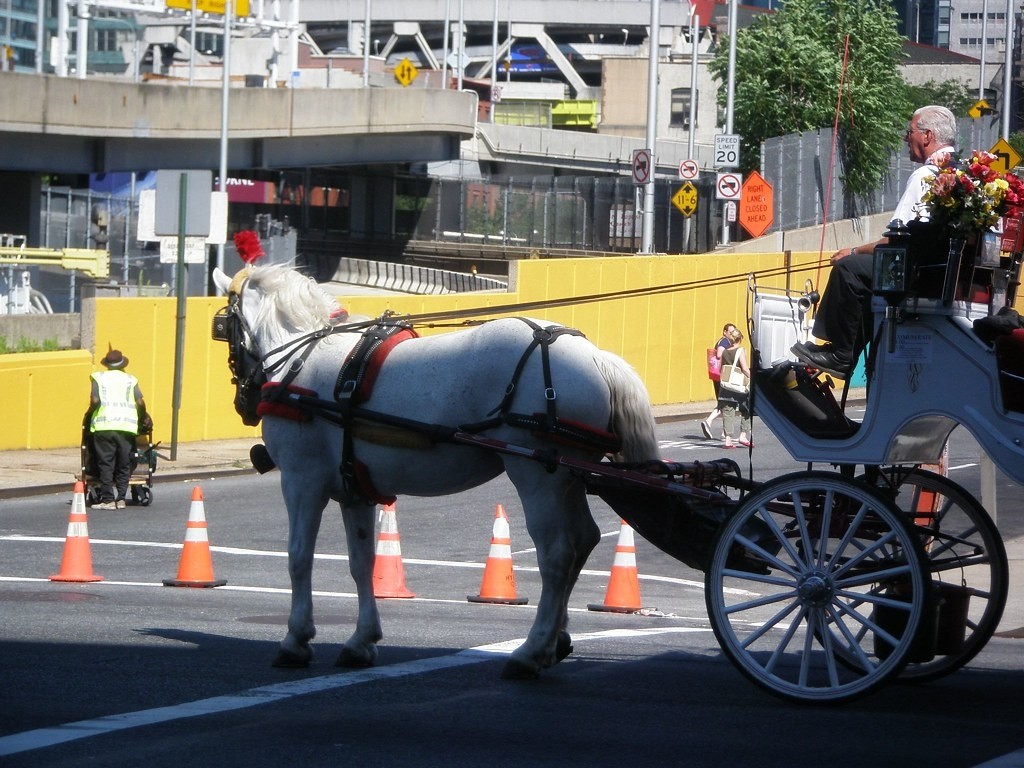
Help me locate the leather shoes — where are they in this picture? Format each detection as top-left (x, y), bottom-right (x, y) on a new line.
top-left (791, 341), bottom-right (860, 380)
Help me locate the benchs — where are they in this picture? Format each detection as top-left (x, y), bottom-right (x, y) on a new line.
top-left (872, 200), bottom-right (1024, 314)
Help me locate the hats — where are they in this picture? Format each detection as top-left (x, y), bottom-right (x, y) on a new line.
top-left (100, 350), bottom-right (129, 369)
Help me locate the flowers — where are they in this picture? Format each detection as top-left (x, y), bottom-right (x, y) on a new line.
top-left (920, 149), bottom-right (1024, 235)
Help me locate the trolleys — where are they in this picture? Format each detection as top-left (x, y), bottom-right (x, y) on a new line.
top-left (80, 425), bottom-right (155, 506)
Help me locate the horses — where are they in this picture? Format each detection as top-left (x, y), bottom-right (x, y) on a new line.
top-left (213, 256), bottom-right (660, 688)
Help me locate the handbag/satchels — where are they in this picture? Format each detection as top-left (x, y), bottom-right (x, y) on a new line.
top-left (707, 337), bottom-right (732, 381)
top-left (719, 347), bottom-right (751, 395)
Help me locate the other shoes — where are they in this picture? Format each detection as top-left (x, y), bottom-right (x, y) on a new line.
top-left (91, 500), bottom-right (117, 509)
top-left (116, 498), bottom-right (126, 509)
top-left (701, 421), bottom-right (713, 440)
top-left (721, 436), bottom-right (733, 441)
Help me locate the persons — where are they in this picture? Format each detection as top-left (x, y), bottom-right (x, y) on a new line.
top-left (701, 324), bottom-right (740, 441)
top-left (718, 329), bottom-right (755, 449)
top-left (790, 105), bottom-right (964, 380)
top-left (90, 350), bottom-right (146, 509)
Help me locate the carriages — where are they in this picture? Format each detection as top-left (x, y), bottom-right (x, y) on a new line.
top-left (209, 147), bottom-right (1024, 713)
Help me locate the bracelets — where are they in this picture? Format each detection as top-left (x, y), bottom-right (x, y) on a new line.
top-left (851, 247), bottom-right (857, 255)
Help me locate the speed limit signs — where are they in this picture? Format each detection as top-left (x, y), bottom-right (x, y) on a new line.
top-left (715, 134), bottom-right (739, 167)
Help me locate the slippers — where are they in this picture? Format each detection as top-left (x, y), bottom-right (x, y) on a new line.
top-left (722, 445), bottom-right (736, 449)
top-left (739, 439), bottom-right (755, 448)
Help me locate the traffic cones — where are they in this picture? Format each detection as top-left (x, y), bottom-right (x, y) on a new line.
top-left (48, 483), bottom-right (104, 582)
top-left (586, 517), bottom-right (660, 615)
top-left (467, 504), bottom-right (529, 605)
top-left (372, 502), bottom-right (418, 597)
top-left (162, 485), bottom-right (228, 589)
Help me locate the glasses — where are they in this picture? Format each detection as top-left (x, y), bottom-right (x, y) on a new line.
top-left (906, 128), bottom-right (932, 136)
